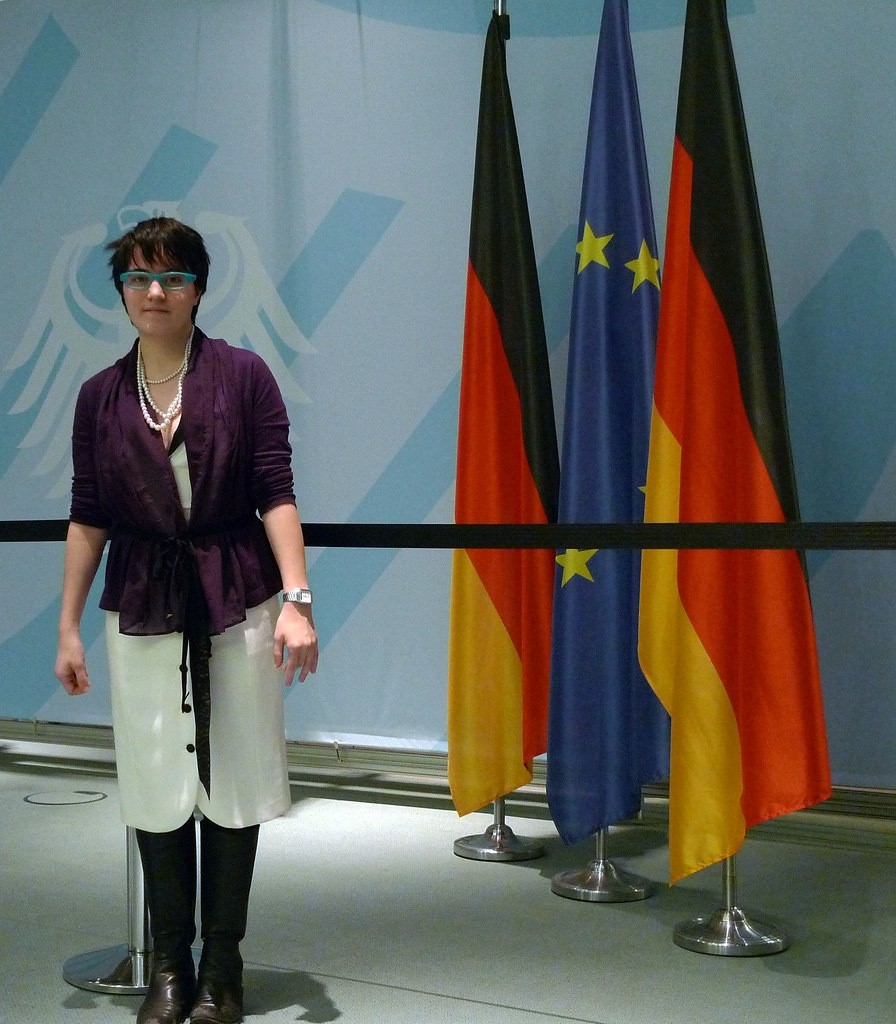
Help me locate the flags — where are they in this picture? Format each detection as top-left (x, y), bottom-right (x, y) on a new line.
top-left (443, 6), bottom-right (568, 822)
top-left (537, 0), bottom-right (676, 850)
top-left (635, 1), bottom-right (837, 891)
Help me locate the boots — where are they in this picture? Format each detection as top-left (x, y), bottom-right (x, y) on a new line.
top-left (136, 819), bottom-right (259, 1024)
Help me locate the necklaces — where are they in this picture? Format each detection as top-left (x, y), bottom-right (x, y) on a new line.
top-left (133, 323), bottom-right (195, 432)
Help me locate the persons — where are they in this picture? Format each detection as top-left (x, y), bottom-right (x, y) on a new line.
top-left (52, 211), bottom-right (320, 1023)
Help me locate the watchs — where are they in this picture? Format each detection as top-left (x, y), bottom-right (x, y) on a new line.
top-left (282, 587), bottom-right (314, 606)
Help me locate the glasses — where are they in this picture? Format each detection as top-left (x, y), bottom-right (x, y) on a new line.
top-left (117, 271), bottom-right (196, 292)
